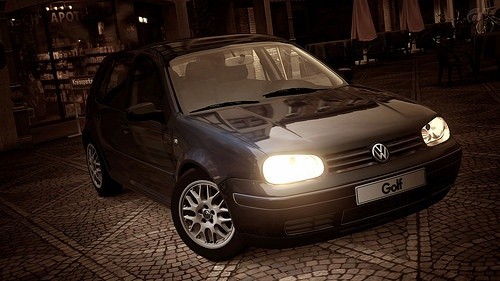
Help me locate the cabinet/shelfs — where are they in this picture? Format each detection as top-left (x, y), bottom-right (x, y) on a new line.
top-left (31, 38), bottom-right (123, 118)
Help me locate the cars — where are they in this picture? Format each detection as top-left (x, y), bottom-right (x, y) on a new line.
top-left (83, 34), bottom-right (463, 262)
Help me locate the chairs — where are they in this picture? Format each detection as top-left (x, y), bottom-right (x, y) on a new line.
top-left (170, 60), bottom-right (261, 113)
top-left (434, 41), bottom-right (473, 88)
